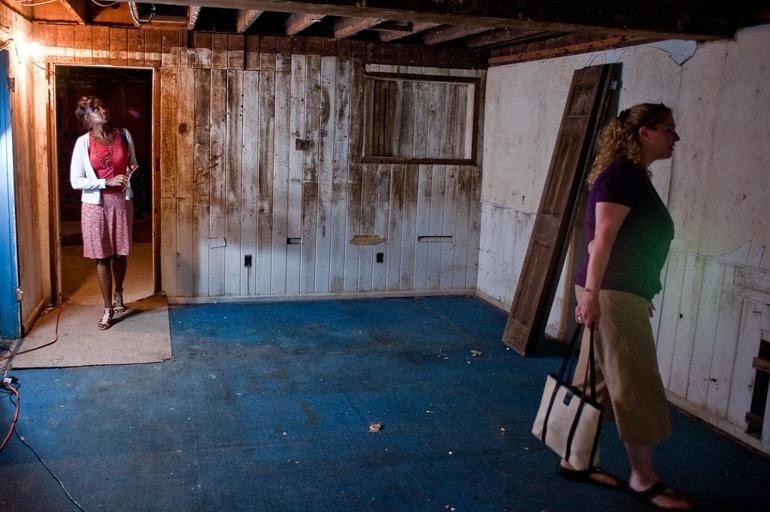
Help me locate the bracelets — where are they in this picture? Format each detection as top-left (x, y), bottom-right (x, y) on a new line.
top-left (581, 287), bottom-right (595, 295)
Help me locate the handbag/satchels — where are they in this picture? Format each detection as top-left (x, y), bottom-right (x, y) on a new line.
top-left (532, 372), bottom-right (603, 471)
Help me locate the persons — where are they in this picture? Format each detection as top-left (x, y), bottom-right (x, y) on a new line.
top-left (69, 93), bottom-right (138, 330)
top-left (569, 104), bottom-right (694, 512)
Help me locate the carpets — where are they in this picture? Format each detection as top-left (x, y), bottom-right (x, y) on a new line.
top-left (7, 289), bottom-right (175, 371)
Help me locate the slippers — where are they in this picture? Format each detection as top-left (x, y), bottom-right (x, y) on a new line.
top-left (557, 464), bottom-right (623, 488)
top-left (626, 481), bottom-right (691, 512)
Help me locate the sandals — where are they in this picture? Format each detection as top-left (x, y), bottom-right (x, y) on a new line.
top-left (97, 307), bottom-right (114, 330)
top-left (113, 288), bottom-right (124, 313)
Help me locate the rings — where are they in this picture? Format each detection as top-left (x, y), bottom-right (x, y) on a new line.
top-left (577, 316), bottom-right (582, 322)
